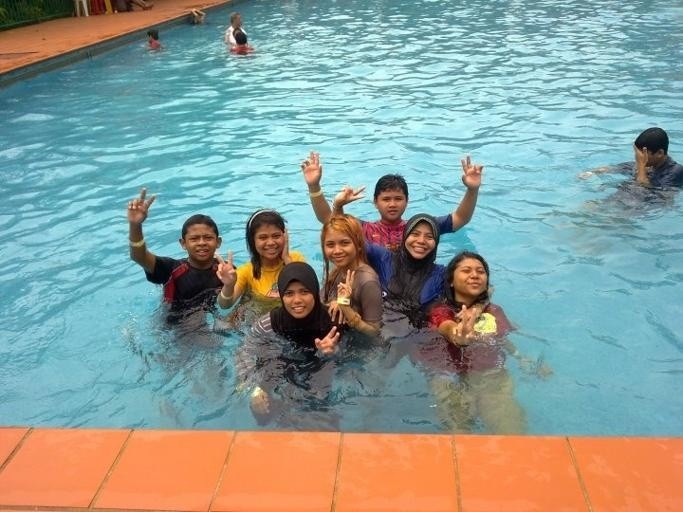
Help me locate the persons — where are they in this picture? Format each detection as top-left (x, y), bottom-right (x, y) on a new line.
top-left (132, 0), bottom-right (154, 10)
top-left (190, 9), bottom-right (206, 26)
top-left (578, 127), bottom-right (683, 255)
top-left (224, 12), bottom-right (255, 53)
top-left (146, 29), bottom-right (161, 49)
